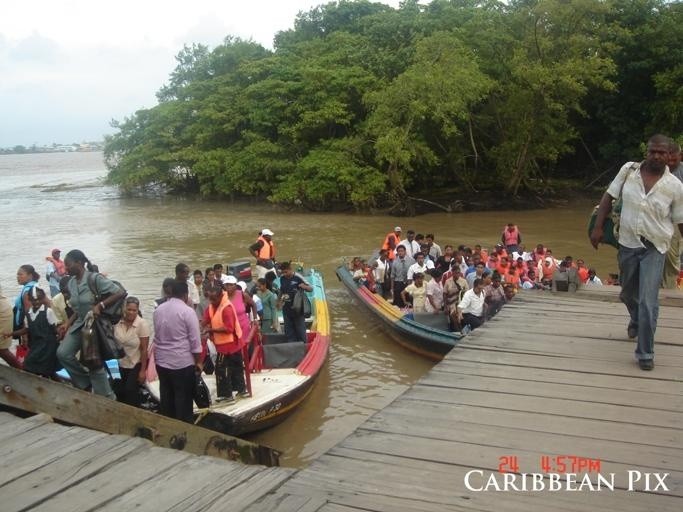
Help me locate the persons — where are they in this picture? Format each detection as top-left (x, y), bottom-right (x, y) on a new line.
top-left (10, 246), bottom-right (315, 422)
top-left (248, 228), bottom-right (276, 266)
top-left (354, 134), bottom-right (683, 371)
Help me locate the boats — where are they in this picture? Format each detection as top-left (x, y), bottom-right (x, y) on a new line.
top-left (0, 260), bottom-right (331, 439)
top-left (336, 244), bottom-right (606, 361)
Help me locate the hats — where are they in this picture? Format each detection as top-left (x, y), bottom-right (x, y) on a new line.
top-left (394, 227), bottom-right (402, 231)
top-left (222, 276), bottom-right (238, 285)
top-left (237, 280), bottom-right (247, 290)
top-left (261, 229), bottom-right (275, 237)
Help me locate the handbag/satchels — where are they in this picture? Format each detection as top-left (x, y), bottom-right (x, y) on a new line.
top-left (96, 281), bottom-right (128, 315)
top-left (588, 197), bottom-right (622, 250)
top-left (203, 347), bottom-right (214, 375)
top-left (146, 342), bottom-right (158, 382)
top-left (291, 287), bottom-right (311, 318)
top-left (79, 327), bottom-right (103, 371)
top-left (194, 378), bottom-right (212, 409)
top-left (16, 345), bottom-right (30, 369)
top-left (94, 317), bottom-right (125, 360)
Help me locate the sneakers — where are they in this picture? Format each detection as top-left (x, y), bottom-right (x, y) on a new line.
top-left (639, 359), bottom-right (654, 370)
top-left (628, 321), bottom-right (638, 338)
